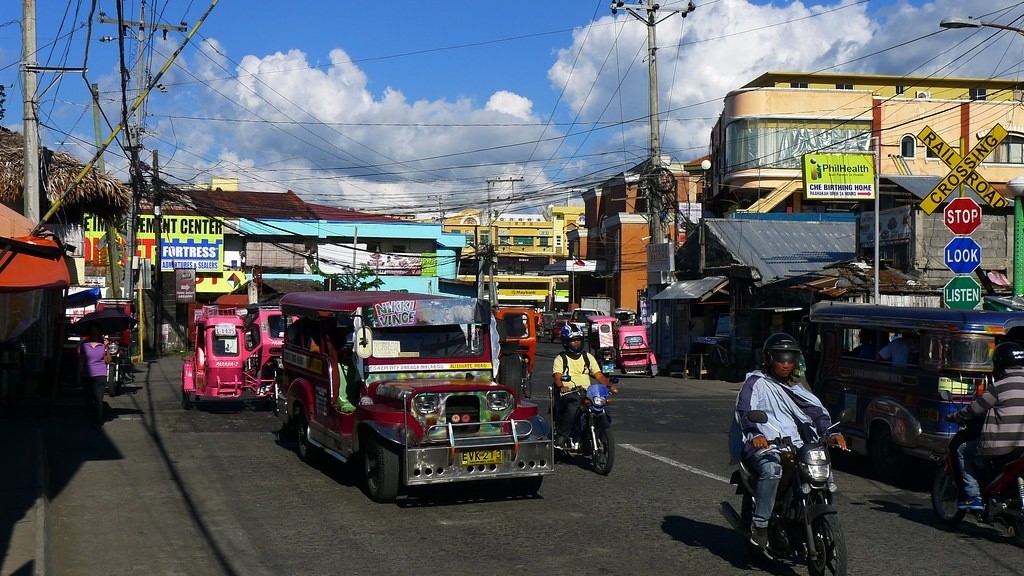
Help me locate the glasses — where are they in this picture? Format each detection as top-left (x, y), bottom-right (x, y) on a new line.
top-left (773, 356), bottom-right (796, 364)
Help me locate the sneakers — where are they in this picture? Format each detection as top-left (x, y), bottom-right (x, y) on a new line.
top-left (554, 435), bottom-right (564, 450)
top-left (750, 522), bottom-right (769, 547)
top-left (957, 496), bottom-right (983, 510)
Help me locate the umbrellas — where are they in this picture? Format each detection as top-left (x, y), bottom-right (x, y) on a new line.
top-left (71, 309), bottom-right (140, 355)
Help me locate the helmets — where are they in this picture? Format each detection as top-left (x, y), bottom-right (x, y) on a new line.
top-left (762, 333), bottom-right (800, 368)
top-left (561, 324), bottom-right (585, 355)
top-left (992, 342), bottom-right (1024, 381)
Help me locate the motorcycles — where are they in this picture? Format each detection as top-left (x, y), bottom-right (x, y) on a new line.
top-left (495, 308), bottom-right (544, 399)
top-left (75, 297), bottom-right (138, 396)
top-left (930, 389), bottom-right (1024, 545)
top-left (181, 305), bottom-right (299, 412)
top-left (717, 406), bottom-right (858, 575)
top-left (587, 316), bottom-right (658, 378)
top-left (547, 376), bottom-right (621, 475)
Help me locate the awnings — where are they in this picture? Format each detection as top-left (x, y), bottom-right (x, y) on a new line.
top-left (651, 278), bottom-right (729, 303)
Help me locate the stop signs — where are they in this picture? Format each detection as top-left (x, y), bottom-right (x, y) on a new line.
top-left (943, 197), bottom-right (983, 236)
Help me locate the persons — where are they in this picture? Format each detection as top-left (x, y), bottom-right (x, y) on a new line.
top-left (78, 326), bottom-right (112, 429)
top-left (728, 332), bottom-right (846, 549)
top-left (552, 325), bottom-right (619, 451)
top-left (812, 326), bottom-right (913, 386)
top-left (945, 342), bottom-right (1023, 510)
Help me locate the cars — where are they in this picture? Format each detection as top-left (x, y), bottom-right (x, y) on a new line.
top-left (792, 298), bottom-right (1024, 472)
top-left (537, 307), bottom-right (638, 343)
top-left (279, 290), bottom-right (551, 503)
